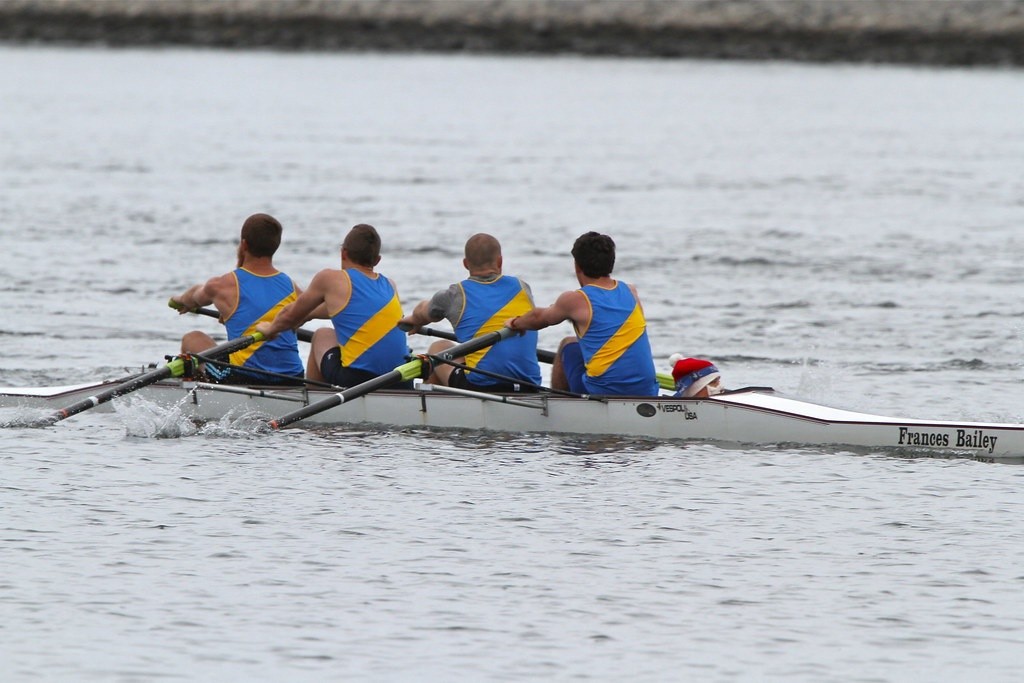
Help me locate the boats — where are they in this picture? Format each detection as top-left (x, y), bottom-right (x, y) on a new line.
top-left (1, 370), bottom-right (1024, 454)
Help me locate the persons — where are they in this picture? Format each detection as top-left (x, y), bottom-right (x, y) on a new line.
top-left (504, 232), bottom-right (660, 395)
top-left (669, 353), bottom-right (722, 397)
top-left (254, 224), bottom-right (409, 389)
top-left (396, 233), bottom-right (543, 389)
top-left (170, 213), bottom-right (305, 385)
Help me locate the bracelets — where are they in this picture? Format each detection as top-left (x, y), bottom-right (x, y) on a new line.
top-left (511, 315), bottom-right (526, 337)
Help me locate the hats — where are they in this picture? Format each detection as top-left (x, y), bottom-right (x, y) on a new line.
top-left (663, 354), bottom-right (720, 399)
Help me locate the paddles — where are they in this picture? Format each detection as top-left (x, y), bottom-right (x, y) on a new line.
top-left (37, 330), bottom-right (264, 426)
top-left (398, 322), bottom-right (678, 392)
top-left (262, 327), bottom-right (516, 433)
top-left (168, 300), bottom-right (314, 343)
